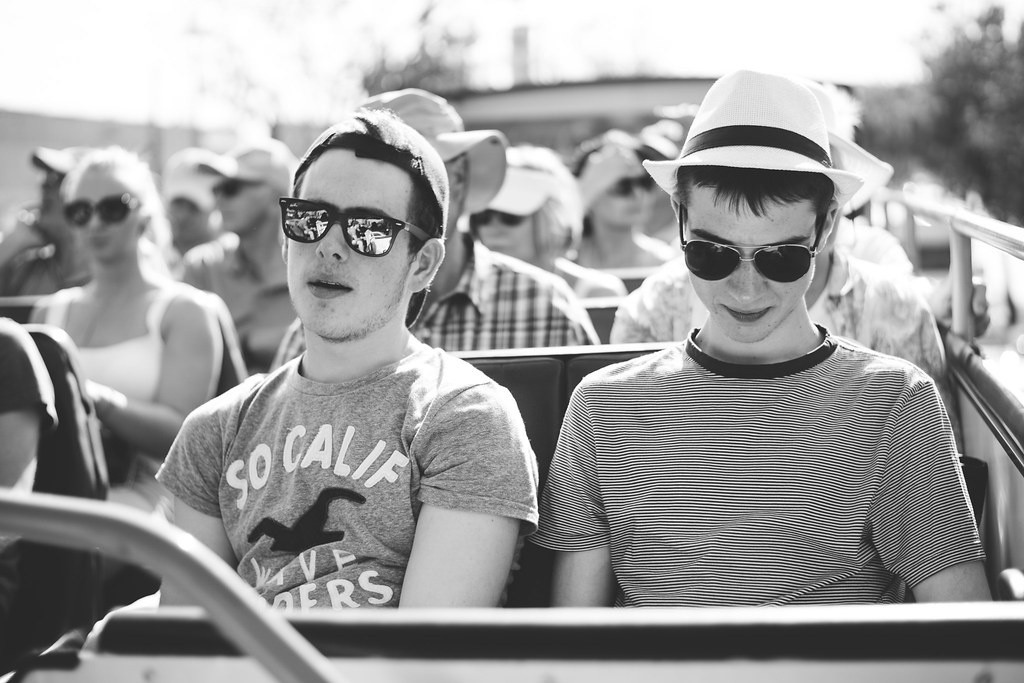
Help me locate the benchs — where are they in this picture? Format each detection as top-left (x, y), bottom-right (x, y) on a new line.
top-left (0, 264), bottom-right (1024, 683)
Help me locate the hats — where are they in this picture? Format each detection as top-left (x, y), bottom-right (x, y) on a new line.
top-left (289, 110), bottom-right (450, 329)
top-left (638, 119), bottom-right (685, 161)
top-left (364, 89), bottom-right (509, 216)
top-left (793, 81), bottom-right (896, 218)
top-left (33, 145), bottom-right (91, 175)
top-left (54, 155), bottom-right (168, 248)
top-left (576, 140), bottom-right (645, 219)
top-left (211, 139), bottom-right (297, 194)
top-left (643, 68), bottom-right (864, 210)
top-left (475, 146), bottom-right (581, 218)
top-left (159, 146), bottom-right (234, 217)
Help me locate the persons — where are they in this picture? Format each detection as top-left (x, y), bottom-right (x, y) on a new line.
top-left (154, 112), bottom-right (540, 608)
top-left (1, 89), bottom-right (963, 669)
top-left (528, 72), bottom-right (995, 606)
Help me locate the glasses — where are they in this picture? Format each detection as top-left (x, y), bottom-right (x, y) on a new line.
top-left (676, 200), bottom-right (840, 282)
top-left (61, 195), bottom-right (132, 225)
top-left (277, 196), bottom-right (430, 257)
top-left (610, 174), bottom-right (656, 198)
